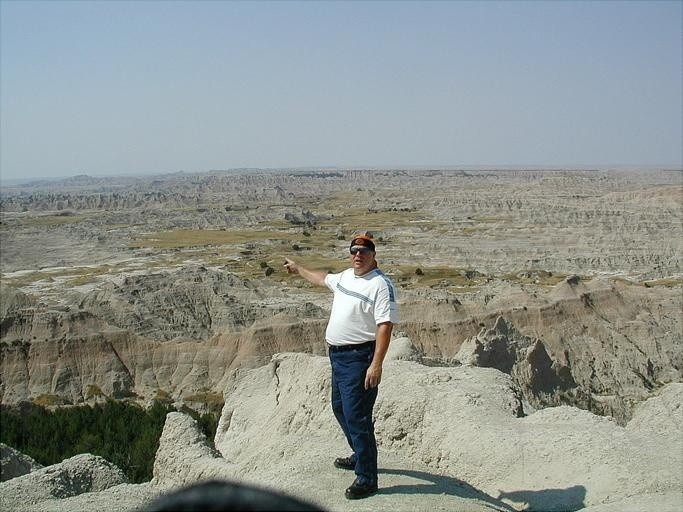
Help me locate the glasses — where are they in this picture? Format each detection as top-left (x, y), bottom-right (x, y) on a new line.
top-left (351, 248), bottom-right (373, 255)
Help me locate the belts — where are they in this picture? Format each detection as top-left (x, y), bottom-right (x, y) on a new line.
top-left (327, 342), bottom-right (376, 351)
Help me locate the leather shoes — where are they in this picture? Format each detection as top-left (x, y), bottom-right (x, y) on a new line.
top-left (346, 481), bottom-right (378, 499)
top-left (334, 458), bottom-right (355, 469)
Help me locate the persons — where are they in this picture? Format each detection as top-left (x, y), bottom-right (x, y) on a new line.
top-left (283, 237), bottom-right (397, 500)
